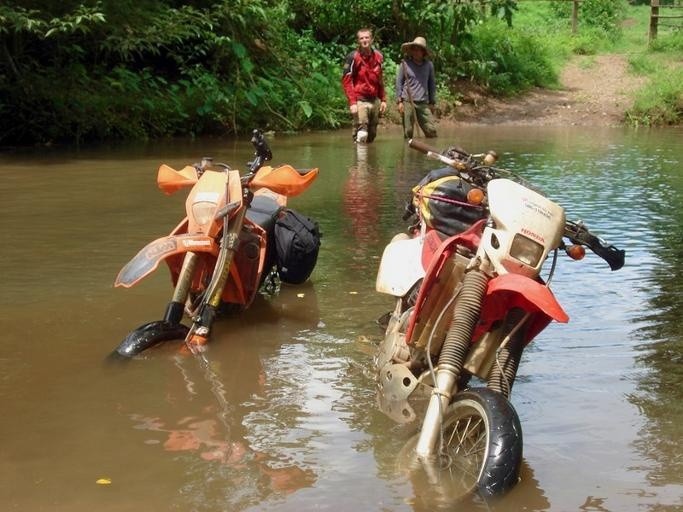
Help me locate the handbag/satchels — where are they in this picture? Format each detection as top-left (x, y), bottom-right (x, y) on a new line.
top-left (274, 207), bottom-right (321, 287)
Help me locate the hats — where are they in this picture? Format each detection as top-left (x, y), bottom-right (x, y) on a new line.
top-left (401, 37), bottom-right (434, 58)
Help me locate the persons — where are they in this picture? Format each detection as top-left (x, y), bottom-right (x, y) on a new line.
top-left (395, 35), bottom-right (439, 140)
top-left (341, 29), bottom-right (387, 144)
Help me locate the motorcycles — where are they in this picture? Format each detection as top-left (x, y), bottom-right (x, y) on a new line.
top-left (103, 129), bottom-right (327, 382)
top-left (129, 342), bottom-right (321, 496)
top-left (376, 139), bottom-right (626, 510)
